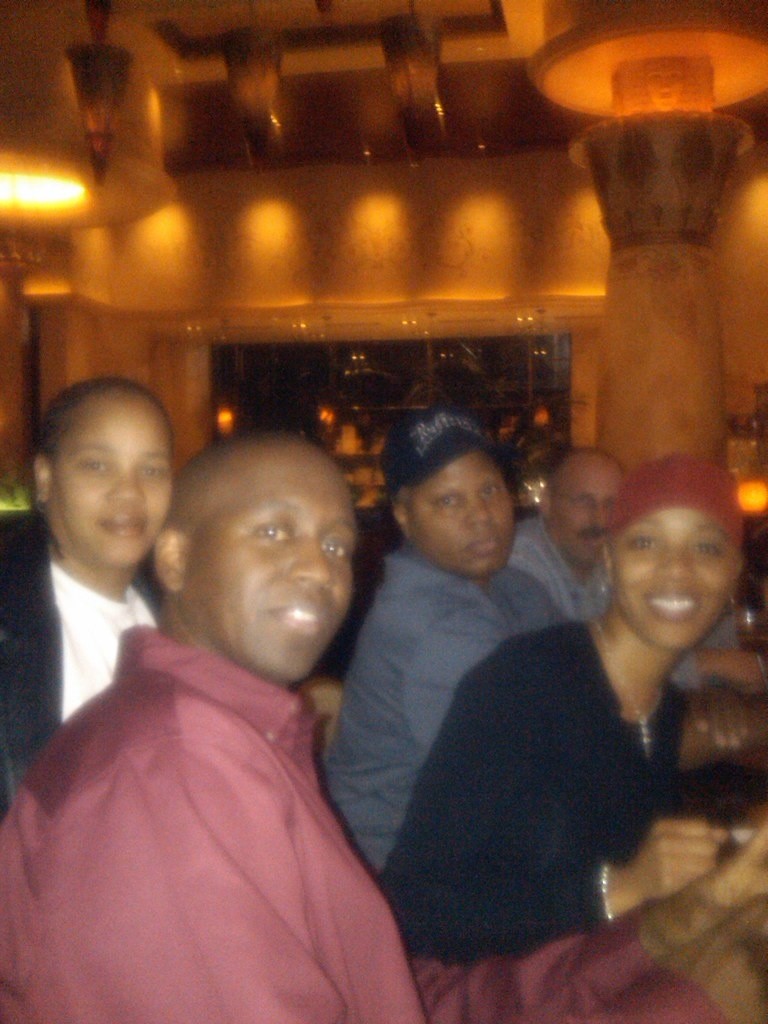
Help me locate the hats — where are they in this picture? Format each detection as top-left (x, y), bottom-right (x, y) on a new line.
top-left (380, 404), bottom-right (504, 496)
top-left (608, 452), bottom-right (744, 550)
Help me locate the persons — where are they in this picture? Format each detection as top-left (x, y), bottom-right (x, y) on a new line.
top-left (0, 377), bottom-right (768, 1024)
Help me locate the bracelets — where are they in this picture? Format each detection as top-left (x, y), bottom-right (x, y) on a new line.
top-left (601, 862), bottom-right (614, 920)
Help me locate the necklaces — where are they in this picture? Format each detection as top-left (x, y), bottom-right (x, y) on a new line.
top-left (594, 616), bottom-right (663, 757)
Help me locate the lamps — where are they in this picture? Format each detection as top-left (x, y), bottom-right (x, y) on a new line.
top-left (64, 0), bottom-right (136, 189)
top-left (222, 27), bottom-right (293, 172)
top-left (380, 8), bottom-right (451, 151)
